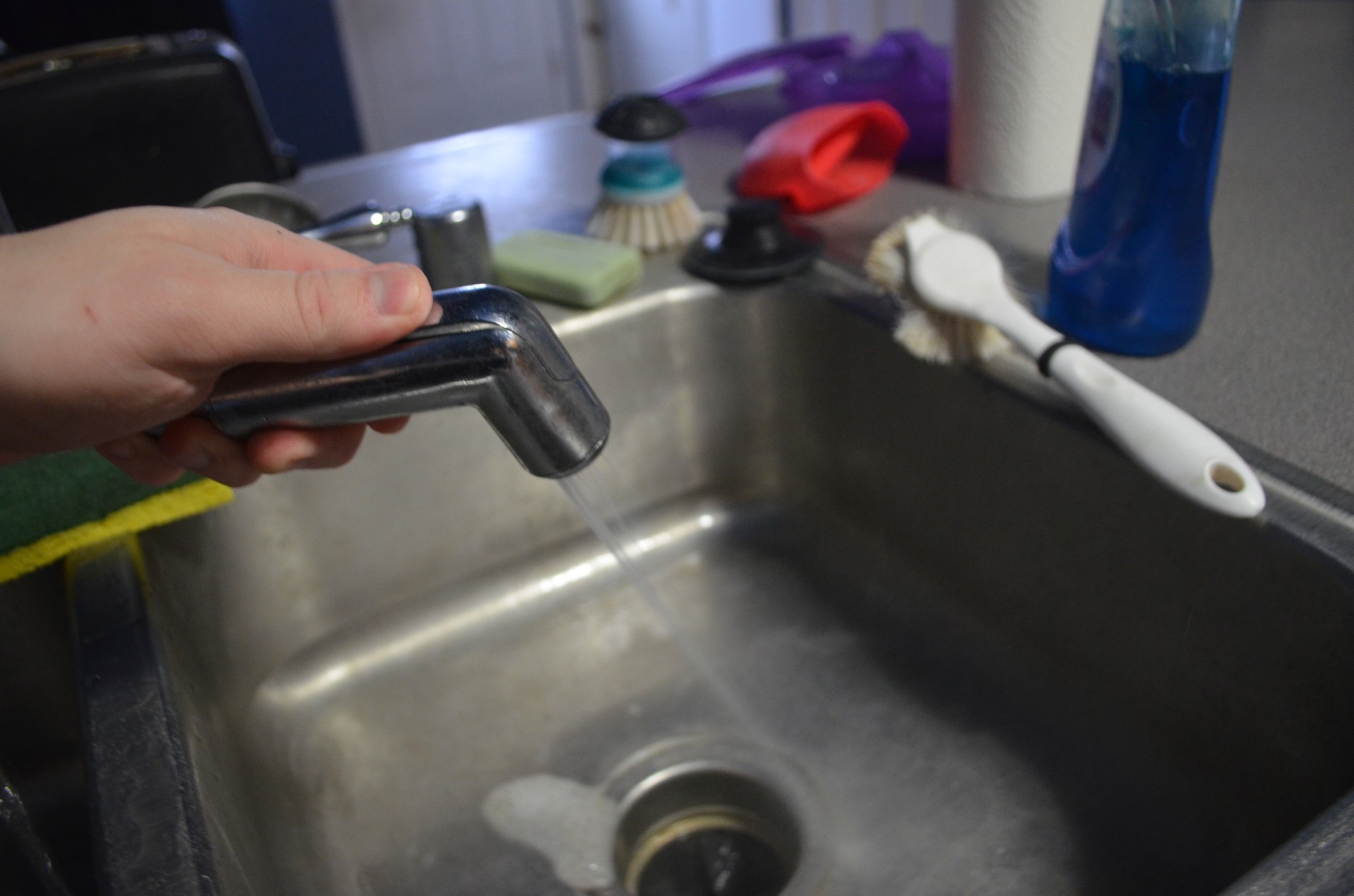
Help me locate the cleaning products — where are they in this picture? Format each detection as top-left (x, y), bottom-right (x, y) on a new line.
top-left (1035, 0), bottom-right (1250, 361)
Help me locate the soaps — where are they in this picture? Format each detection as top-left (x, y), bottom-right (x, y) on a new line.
top-left (475, 225), bottom-right (649, 310)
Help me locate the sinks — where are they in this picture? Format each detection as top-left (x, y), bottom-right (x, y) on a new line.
top-left (21, 219), bottom-right (1354, 895)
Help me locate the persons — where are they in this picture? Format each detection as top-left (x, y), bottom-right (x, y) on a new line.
top-left (0, 202), bottom-right (437, 493)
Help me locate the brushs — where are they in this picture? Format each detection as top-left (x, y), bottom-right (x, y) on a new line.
top-left (584, 152), bottom-right (703, 254)
top-left (591, 36), bottom-right (853, 161)
top-left (867, 208), bottom-right (1266, 522)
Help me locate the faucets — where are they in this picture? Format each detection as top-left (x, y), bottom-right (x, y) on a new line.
top-left (193, 283), bottom-right (616, 484)
